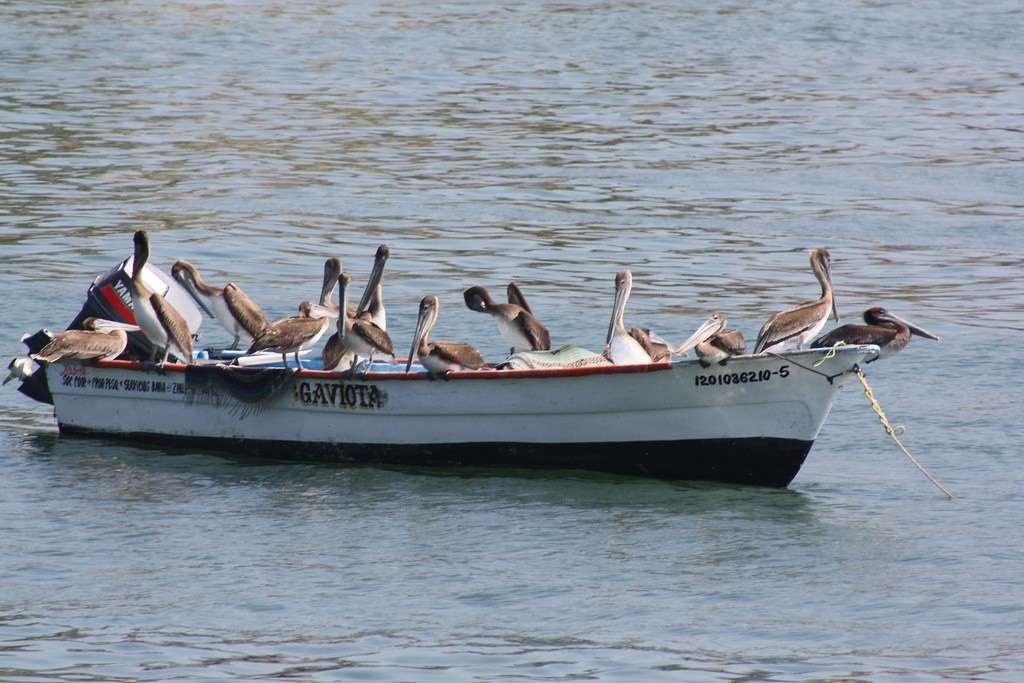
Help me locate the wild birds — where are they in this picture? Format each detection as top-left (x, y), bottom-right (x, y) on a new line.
top-left (336, 271), bottom-right (396, 372)
top-left (298, 258), bottom-right (359, 318)
top-left (30, 317), bottom-right (143, 363)
top-left (244, 301), bottom-right (337, 372)
top-left (405, 295), bottom-right (486, 372)
top-left (602, 270), bottom-right (670, 366)
top-left (131, 229), bottom-right (194, 369)
top-left (627, 326), bottom-right (689, 363)
top-left (171, 260), bottom-right (268, 349)
top-left (675, 312), bottom-right (747, 364)
top-left (322, 244), bottom-right (390, 371)
top-left (464, 282), bottom-right (551, 351)
top-left (754, 247), bottom-right (840, 353)
top-left (810, 307), bottom-right (940, 358)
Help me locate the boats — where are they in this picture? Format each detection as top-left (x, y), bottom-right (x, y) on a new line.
top-left (16, 252), bottom-right (884, 490)
